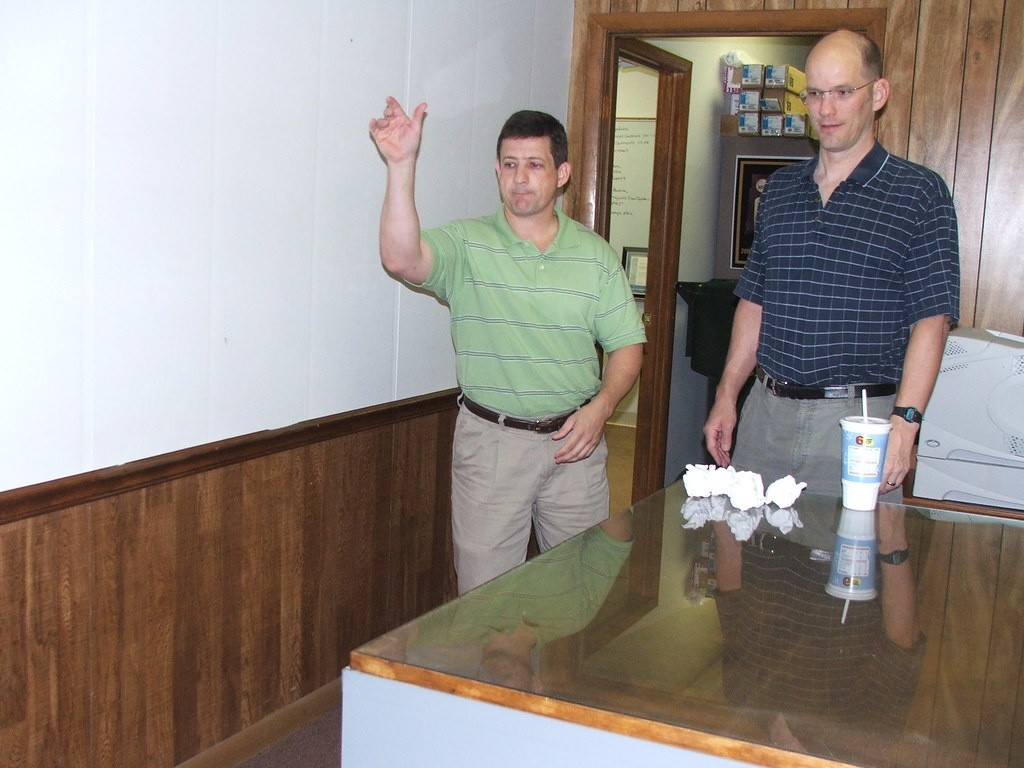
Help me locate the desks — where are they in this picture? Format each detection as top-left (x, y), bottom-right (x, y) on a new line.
top-left (342, 469), bottom-right (1023, 768)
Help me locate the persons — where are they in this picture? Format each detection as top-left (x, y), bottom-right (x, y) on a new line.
top-left (369, 96), bottom-right (649, 598)
top-left (707, 506), bottom-right (928, 768)
top-left (372, 508), bottom-right (637, 695)
top-left (703, 29), bottom-right (962, 505)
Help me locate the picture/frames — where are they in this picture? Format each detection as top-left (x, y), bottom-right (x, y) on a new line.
top-left (729, 155), bottom-right (816, 269)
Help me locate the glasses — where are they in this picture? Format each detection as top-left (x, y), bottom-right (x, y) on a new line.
top-left (799, 80), bottom-right (875, 107)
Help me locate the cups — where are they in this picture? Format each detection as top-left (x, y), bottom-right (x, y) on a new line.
top-left (839, 416), bottom-right (894, 512)
top-left (824, 506), bottom-right (881, 601)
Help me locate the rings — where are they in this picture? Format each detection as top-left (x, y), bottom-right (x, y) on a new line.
top-left (887, 481), bottom-right (895, 486)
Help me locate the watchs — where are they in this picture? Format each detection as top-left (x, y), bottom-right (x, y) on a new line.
top-left (878, 543), bottom-right (910, 565)
top-left (891, 406), bottom-right (922, 424)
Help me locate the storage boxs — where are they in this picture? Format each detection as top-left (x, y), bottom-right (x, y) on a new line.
top-left (719, 63), bottom-right (815, 139)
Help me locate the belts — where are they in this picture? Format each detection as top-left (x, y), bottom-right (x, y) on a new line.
top-left (756, 365), bottom-right (895, 399)
top-left (463, 395), bottom-right (592, 434)
top-left (743, 531), bottom-right (834, 565)
